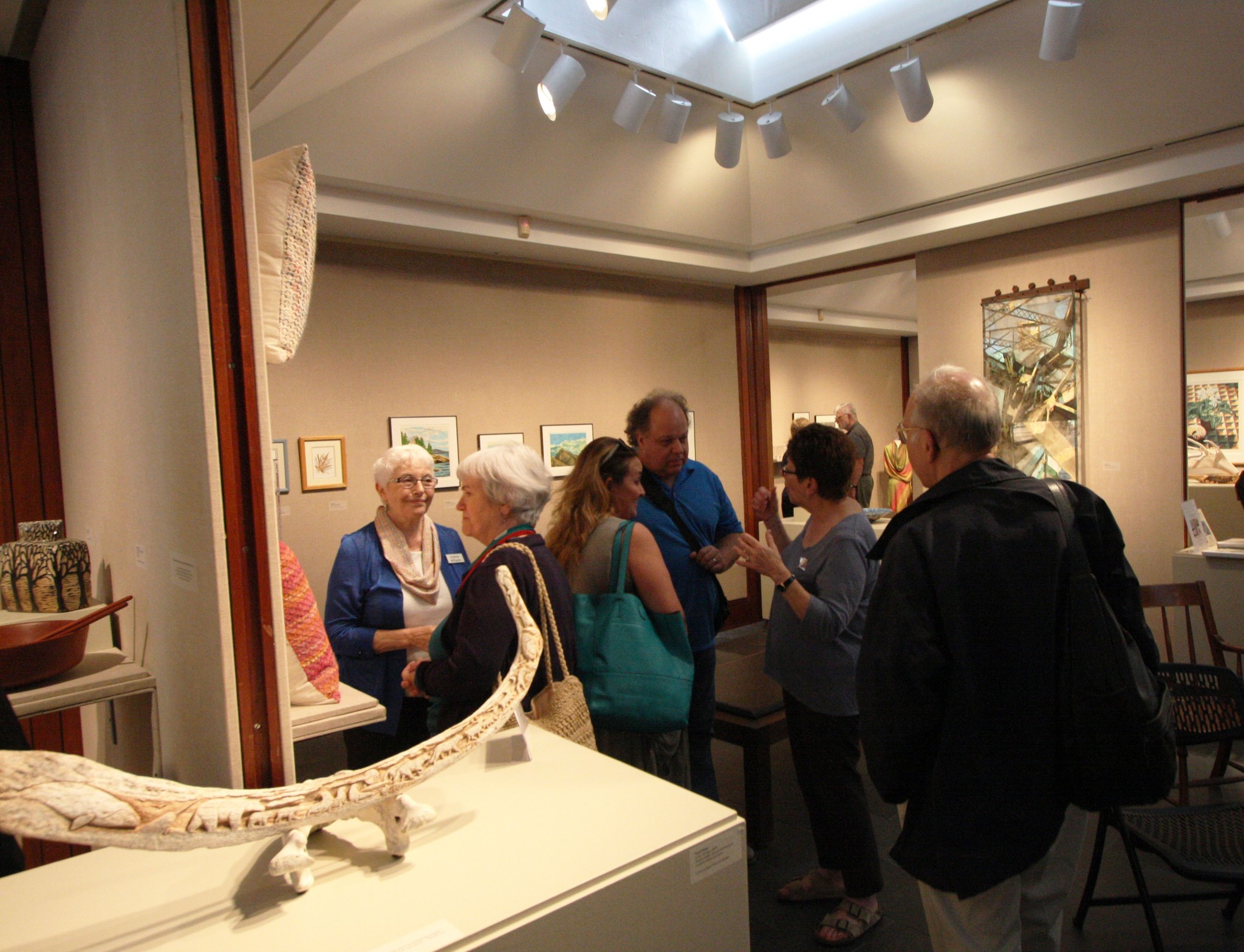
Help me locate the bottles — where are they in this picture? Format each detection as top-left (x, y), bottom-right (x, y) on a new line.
top-left (1, 519), bottom-right (91, 614)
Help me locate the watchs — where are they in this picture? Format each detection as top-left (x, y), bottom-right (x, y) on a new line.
top-left (776, 574), bottom-right (796, 592)
top-left (849, 484), bottom-right (857, 488)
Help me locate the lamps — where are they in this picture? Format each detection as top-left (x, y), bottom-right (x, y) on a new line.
top-left (889, 42), bottom-right (934, 123)
top-left (714, 97), bottom-right (744, 168)
top-left (494, 1), bottom-right (545, 74)
top-left (656, 76), bottom-right (692, 145)
top-left (1038, 1), bottom-right (1083, 63)
top-left (537, 39), bottom-right (585, 121)
top-left (757, 97), bottom-right (794, 161)
top-left (587, 0), bottom-right (616, 19)
top-left (822, 70), bottom-right (867, 132)
top-left (614, 64), bottom-right (655, 135)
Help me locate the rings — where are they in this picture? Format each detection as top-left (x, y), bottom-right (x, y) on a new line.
top-left (746, 556), bottom-right (749, 561)
top-left (713, 558), bottom-right (717, 564)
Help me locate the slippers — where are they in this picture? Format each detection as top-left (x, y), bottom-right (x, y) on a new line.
top-left (775, 871), bottom-right (848, 903)
top-left (815, 900), bottom-right (882, 947)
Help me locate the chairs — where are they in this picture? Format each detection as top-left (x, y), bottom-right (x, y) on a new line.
top-left (1072, 580), bottom-right (1244, 952)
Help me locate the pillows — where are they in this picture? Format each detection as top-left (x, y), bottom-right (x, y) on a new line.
top-left (280, 539), bottom-right (342, 707)
top-left (253, 145), bottom-right (317, 365)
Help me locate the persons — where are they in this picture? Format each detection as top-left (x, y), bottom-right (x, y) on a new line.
top-left (883, 438), bottom-right (913, 516)
top-left (781, 418), bottom-right (810, 518)
top-left (544, 437), bottom-right (690, 794)
top-left (834, 404), bottom-right (874, 508)
top-left (402, 443), bottom-right (570, 738)
top-left (855, 366), bottom-right (1157, 952)
top-left (324, 443), bottom-right (471, 771)
top-left (624, 389), bottom-right (745, 802)
top-left (734, 422), bottom-right (885, 952)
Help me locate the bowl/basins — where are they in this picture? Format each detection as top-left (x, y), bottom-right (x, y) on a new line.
top-left (0, 620), bottom-right (89, 686)
top-left (863, 507), bottom-right (893, 523)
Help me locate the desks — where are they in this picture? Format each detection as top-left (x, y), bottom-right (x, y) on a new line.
top-left (7, 646), bottom-right (163, 779)
top-left (290, 681), bottom-right (386, 744)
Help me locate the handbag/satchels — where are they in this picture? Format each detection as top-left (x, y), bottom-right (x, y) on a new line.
top-left (1047, 484), bottom-right (1179, 811)
top-left (501, 675), bottom-right (597, 750)
top-left (571, 519), bottom-right (693, 731)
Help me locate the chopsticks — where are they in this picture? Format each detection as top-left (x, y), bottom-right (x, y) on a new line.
top-left (34, 596), bottom-right (133, 640)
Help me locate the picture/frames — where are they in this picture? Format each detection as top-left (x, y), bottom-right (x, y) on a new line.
top-left (792, 412), bottom-right (809, 420)
top-left (815, 414), bottom-right (841, 430)
top-left (477, 433), bottom-right (524, 450)
top-left (388, 415), bottom-right (461, 489)
top-left (540, 423), bottom-right (593, 479)
top-left (1186, 367), bottom-right (1244, 467)
top-left (686, 411), bottom-right (695, 461)
top-left (273, 439), bottom-right (290, 494)
top-left (298, 435), bottom-right (348, 492)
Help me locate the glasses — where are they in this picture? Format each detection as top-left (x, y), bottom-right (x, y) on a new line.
top-left (897, 424), bottom-right (940, 451)
top-left (781, 466), bottom-right (812, 479)
top-left (836, 412), bottom-right (849, 421)
top-left (390, 474), bottom-right (438, 490)
